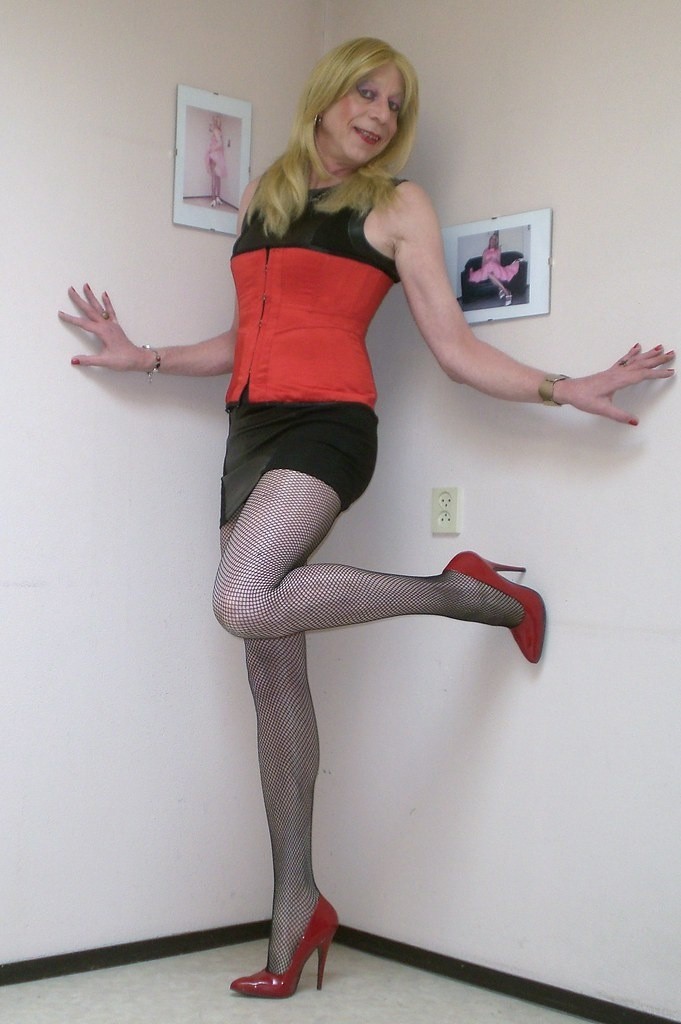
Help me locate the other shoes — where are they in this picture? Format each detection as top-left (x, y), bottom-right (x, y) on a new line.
top-left (499, 290), bottom-right (505, 299)
top-left (505, 293), bottom-right (513, 305)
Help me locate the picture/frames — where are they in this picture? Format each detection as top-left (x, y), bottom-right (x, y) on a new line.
top-left (441, 208), bottom-right (553, 323)
top-left (173, 83), bottom-right (252, 235)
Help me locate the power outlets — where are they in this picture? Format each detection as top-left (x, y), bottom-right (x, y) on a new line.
top-left (430, 487), bottom-right (461, 534)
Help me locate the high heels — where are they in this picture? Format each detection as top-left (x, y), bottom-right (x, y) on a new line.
top-left (443, 552), bottom-right (546, 664)
top-left (211, 200), bottom-right (215, 207)
top-left (216, 197), bottom-right (223, 205)
top-left (231, 895), bottom-right (339, 998)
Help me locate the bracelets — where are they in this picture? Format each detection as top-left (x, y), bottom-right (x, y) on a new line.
top-left (141, 343), bottom-right (162, 383)
top-left (539, 374), bottom-right (570, 407)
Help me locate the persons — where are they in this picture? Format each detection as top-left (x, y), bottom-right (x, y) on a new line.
top-left (59, 37), bottom-right (674, 1002)
top-left (468, 234), bottom-right (520, 306)
top-left (207, 116), bottom-right (225, 207)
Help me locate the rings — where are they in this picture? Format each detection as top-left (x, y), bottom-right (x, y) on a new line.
top-left (102, 311), bottom-right (109, 320)
top-left (619, 360), bottom-right (629, 367)
